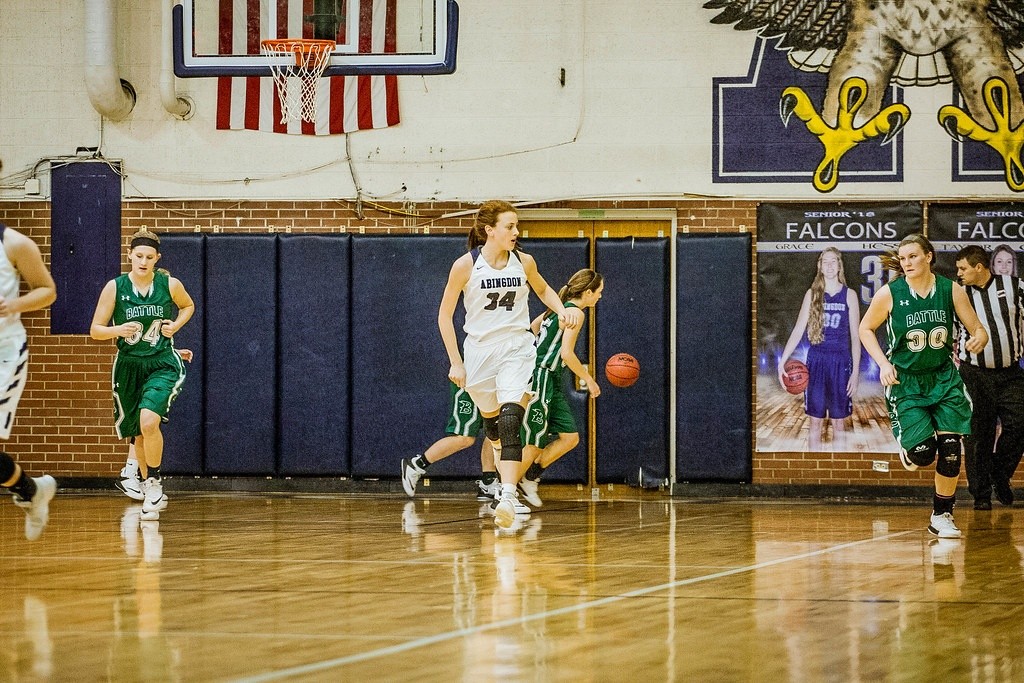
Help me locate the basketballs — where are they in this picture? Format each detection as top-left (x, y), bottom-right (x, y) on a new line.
top-left (783, 360), bottom-right (808, 394)
top-left (605, 353), bottom-right (640, 389)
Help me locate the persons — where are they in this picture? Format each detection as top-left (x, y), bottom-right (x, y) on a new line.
top-left (90, 231), bottom-right (195, 513)
top-left (114, 267), bottom-right (194, 500)
top-left (858, 235), bottom-right (989, 538)
top-left (953, 245), bottom-right (1024, 510)
top-left (0, 157), bottom-right (58, 540)
top-left (778, 247), bottom-right (862, 453)
top-left (990, 244), bottom-right (1018, 278)
top-left (438, 199), bottom-right (577, 515)
top-left (514, 268), bottom-right (600, 515)
top-left (400, 334), bottom-right (501, 503)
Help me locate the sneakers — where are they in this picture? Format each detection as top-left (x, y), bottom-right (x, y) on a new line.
top-left (990, 453), bottom-right (1014, 506)
top-left (927, 509), bottom-right (962, 539)
top-left (974, 497), bottom-right (993, 510)
top-left (494, 497), bottom-right (517, 528)
top-left (898, 445), bottom-right (920, 472)
top-left (115, 467), bottom-right (145, 501)
top-left (475, 478), bottom-right (520, 502)
top-left (141, 476), bottom-right (163, 520)
top-left (490, 496), bottom-right (531, 513)
top-left (12, 474), bottom-right (57, 541)
top-left (401, 453), bottom-right (426, 497)
top-left (516, 477), bottom-right (543, 508)
top-left (139, 480), bottom-right (169, 501)
